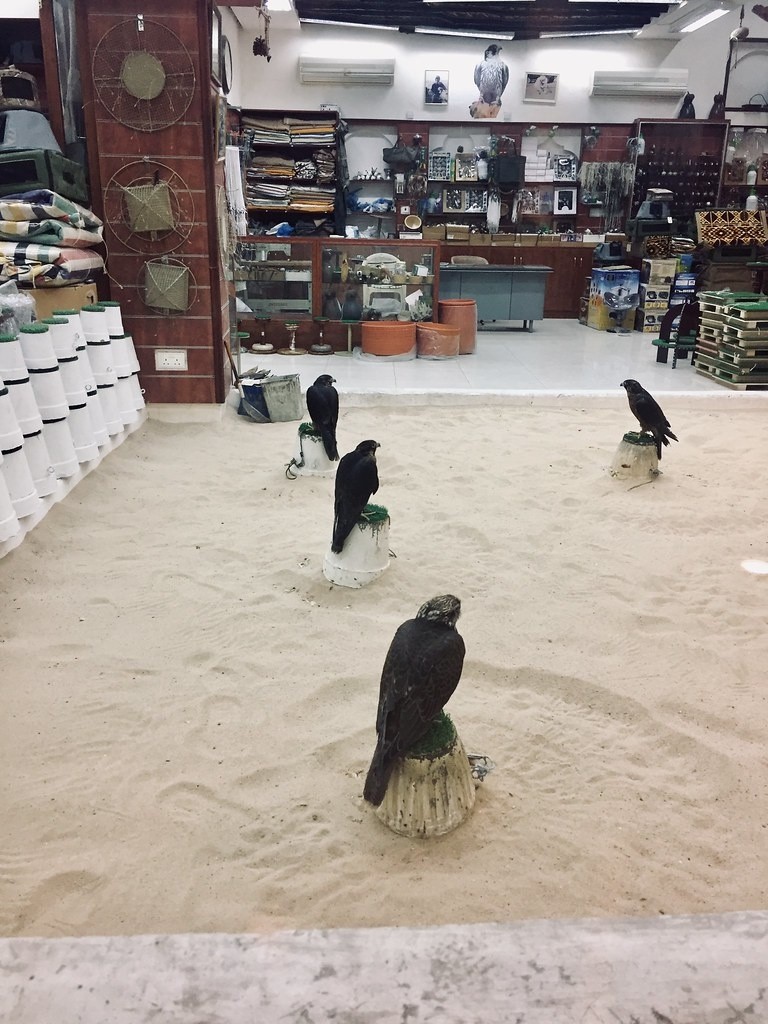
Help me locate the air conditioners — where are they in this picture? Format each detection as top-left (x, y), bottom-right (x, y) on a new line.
top-left (298, 54), bottom-right (396, 86)
top-left (588, 67), bottom-right (689, 100)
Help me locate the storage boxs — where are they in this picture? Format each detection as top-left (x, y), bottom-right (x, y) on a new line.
top-left (638, 283), bottom-right (671, 310)
top-left (634, 308), bottom-right (668, 332)
top-left (672, 253), bottom-right (695, 272)
top-left (421, 224), bottom-right (626, 242)
top-left (641, 259), bottom-right (677, 285)
top-left (554, 187), bottom-right (577, 214)
top-left (455, 152), bottom-right (477, 181)
top-left (586, 268), bottom-right (640, 331)
top-left (442, 188), bottom-right (465, 212)
top-left (667, 298), bottom-right (691, 331)
top-left (670, 273), bottom-right (696, 300)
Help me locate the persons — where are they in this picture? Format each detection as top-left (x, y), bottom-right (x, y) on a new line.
top-left (431, 77), bottom-right (447, 103)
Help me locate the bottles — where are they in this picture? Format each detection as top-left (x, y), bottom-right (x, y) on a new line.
top-left (746, 165), bottom-right (757, 185)
top-left (637, 133), bottom-right (645, 155)
top-left (745, 190), bottom-right (758, 211)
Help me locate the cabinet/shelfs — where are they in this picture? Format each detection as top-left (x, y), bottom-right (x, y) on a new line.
top-left (232, 235), bottom-right (441, 350)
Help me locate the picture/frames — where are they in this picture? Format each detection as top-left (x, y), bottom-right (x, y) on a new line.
top-left (523, 72), bottom-right (559, 103)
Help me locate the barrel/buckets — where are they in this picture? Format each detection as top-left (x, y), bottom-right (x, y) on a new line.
top-left (0, 301), bottom-right (146, 543)
top-left (607, 431), bottom-right (660, 483)
top-left (373, 713), bottom-right (476, 840)
top-left (323, 504), bottom-right (397, 590)
top-left (289, 423), bottom-right (342, 481)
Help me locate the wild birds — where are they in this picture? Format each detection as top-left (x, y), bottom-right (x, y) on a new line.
top-left (329, 438), bottom-right (381, 555)
top-left (361, 594), bottom-right (467, 807)
top-left (305, 374), bottom-right (340, 461)
top-left (620, 379), bottom-right (679, 460)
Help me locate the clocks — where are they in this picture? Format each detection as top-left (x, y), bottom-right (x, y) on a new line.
top-left (220, 35), bottom-right (233, 94)
